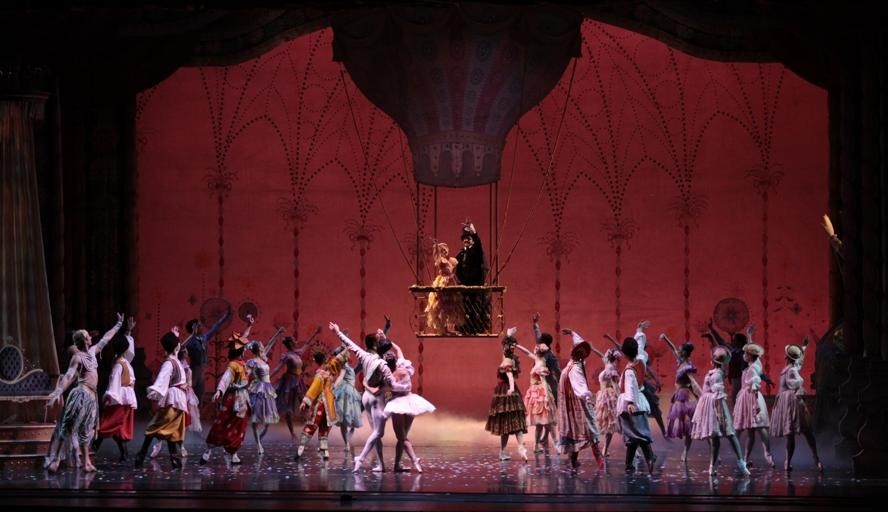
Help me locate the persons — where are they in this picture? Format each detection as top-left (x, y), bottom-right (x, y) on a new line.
top-left (484, 310), bottom-right (825, 476)
top-left (45, 311), bottom-right (435, 474)
top-left (456, 216), bottom-right (493, 333)
top-left (424, 237), bottom-right (458, 335)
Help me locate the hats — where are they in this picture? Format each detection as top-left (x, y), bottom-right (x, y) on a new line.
top-left (785, 344), bottom-right (805, 360)
top-left (162, 332), bottom-right (179, 353)
top-left (710, 346), bottom-right (732, 365)
top-left (742, 343), bottom-right (764, 355)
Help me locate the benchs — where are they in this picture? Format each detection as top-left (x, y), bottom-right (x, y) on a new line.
top-left (1, 341), bottom-right (64, 423)
